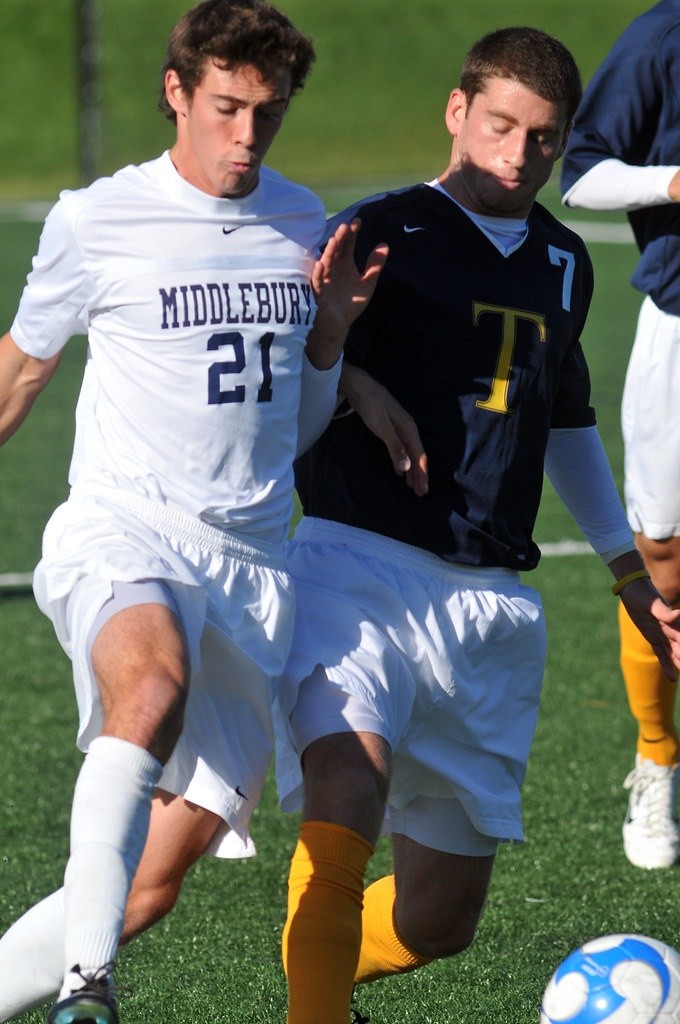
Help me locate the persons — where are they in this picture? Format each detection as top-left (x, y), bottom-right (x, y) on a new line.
top-left (0, 0), bottom-right (432, 1024)
top-left (560, 1), bottom-right (680, 869)
top-left (272, 28), bottom-right (680, 1024)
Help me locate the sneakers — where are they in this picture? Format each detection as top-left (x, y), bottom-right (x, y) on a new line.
top-left (47, 961), bottom-right (133, 1024)
top-left (621, 753), bottom-right (680, 871)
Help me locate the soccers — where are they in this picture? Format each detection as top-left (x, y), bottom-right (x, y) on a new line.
top-left (540, 932), bottom-right (680, 1024)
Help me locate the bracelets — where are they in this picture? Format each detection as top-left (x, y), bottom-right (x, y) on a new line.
top-left (611, 571), bottom-right (651, 596)
